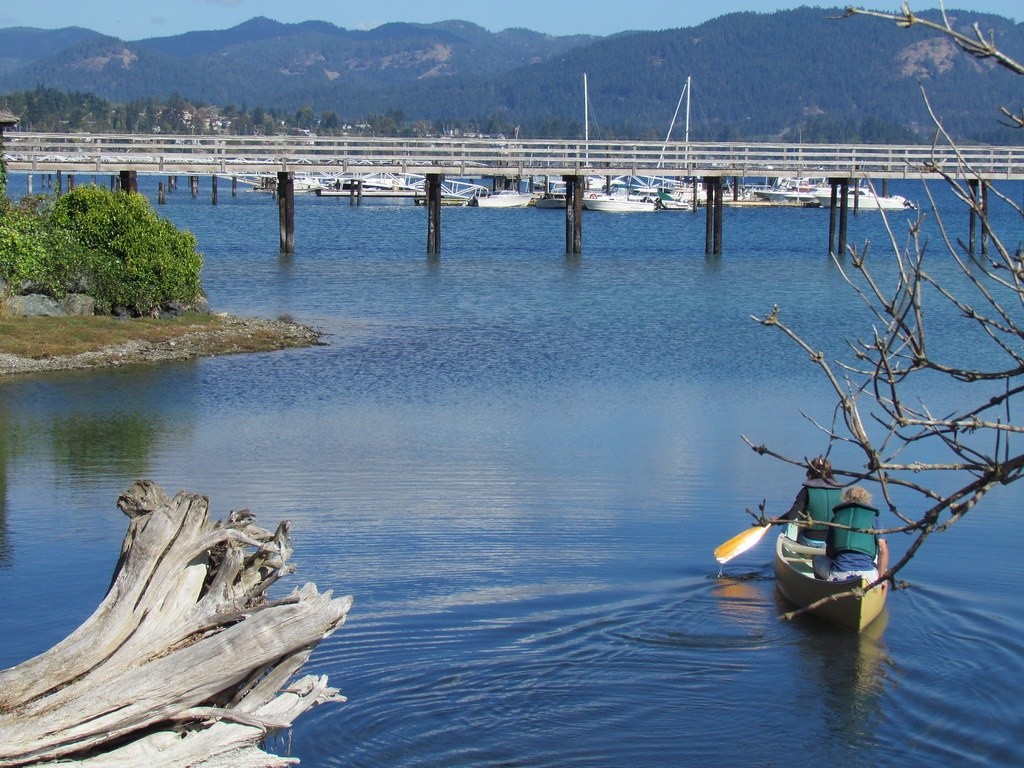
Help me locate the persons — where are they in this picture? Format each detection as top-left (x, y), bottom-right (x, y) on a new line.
top-left (770, 457), bottom-right (844, 559)
top-left (812, 485), bottom-right (889, 590)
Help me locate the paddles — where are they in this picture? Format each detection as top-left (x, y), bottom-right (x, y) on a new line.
top-left (713, 516), bottom-right (775, 567)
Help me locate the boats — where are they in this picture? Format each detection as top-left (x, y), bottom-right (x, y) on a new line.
top-left (476, 188), bottom-right (533, 209)
top-left (413, 196), bottom-right (468, 207)
top-left (814, 185), bottom-right (915, 209)
top-left (774, 530), bottom-right (889, 632)
top-left (244, 174), bottom-right (444, 198)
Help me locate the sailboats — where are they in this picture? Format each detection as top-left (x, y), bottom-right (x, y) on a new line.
top-left (533, 71), bottom-right (752, 213)
top-left (753, 128), bottom-right (821, 205)
